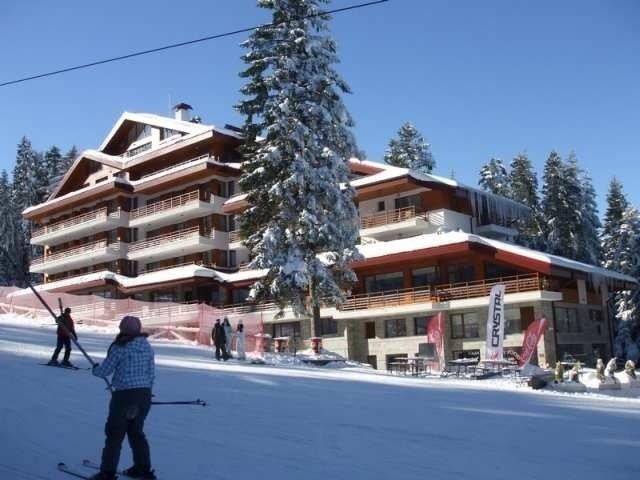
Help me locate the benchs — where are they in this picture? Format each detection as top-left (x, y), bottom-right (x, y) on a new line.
top-left (469, 366), bottom-right (490, 376)
top-left (514, 369), bottom-right (520, 376)
top-left (423, 362), bottom-right (433, 375)
top-left (388, 362), bottom-right (415, 376)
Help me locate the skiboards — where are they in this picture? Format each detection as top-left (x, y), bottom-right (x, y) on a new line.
top-left (58, 460), bottom-right (157, 480)
top-left (37, 362), bottom-right (91, 370)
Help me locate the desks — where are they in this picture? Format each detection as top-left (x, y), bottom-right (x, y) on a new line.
top-left (556, 362), bottom-right (586, 381)
top-left (447, 360), bottom-right (478, 375)
top-left (480, 359), bottom-right (513, 375)
top-left (394, 356), bottom-right (434, 377)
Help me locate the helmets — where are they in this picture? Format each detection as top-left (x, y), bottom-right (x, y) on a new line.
top-left (119, 316), bottom-right (141, 336)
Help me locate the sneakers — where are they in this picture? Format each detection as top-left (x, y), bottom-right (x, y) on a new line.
top-left (123, 464), bottom-right (150, 476)
top-left (94, 469), bottom-right (115, 480)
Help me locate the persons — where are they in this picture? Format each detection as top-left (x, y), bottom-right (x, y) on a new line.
top-left (221, 315), bottom-right (234, 358)
top-left (235, 319), bottom-right (246, 360)
top-left (51, 307), bottom-right (77, 364)
top-left (211, 319), bottom-right (228, 361)
top-left (91, 316), bottom-right (155, 480)
